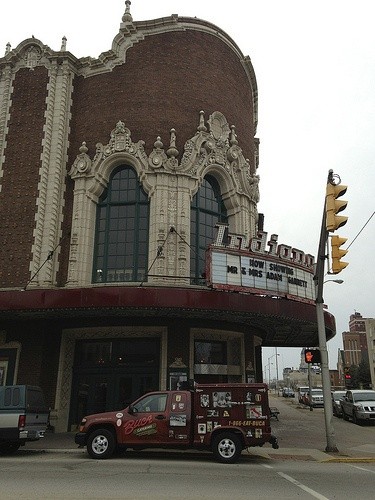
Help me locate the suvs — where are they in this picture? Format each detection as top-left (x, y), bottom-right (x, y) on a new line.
top-left (0, 385), bottom-right (50, 454)
top-left (339, 390), bottom-right (375, 425)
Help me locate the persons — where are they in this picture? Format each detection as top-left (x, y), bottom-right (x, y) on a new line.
top-left (201, 392), bottom-right (231, 407)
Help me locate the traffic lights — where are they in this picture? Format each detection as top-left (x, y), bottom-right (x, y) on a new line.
top-left (342, 365), bottom-right (351, 379)
top-left (326, 182), bottom-right (349, 232)
top-left (331, 234), bottom-right (349, 274)
top-left (304, 349), bottom-right (321, 364)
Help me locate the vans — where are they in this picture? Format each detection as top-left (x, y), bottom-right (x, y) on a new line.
top-left (298, 386), bottom-right (309, 404)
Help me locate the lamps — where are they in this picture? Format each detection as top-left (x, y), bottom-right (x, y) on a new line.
top-left (323, 280), bottom-right (344, 284)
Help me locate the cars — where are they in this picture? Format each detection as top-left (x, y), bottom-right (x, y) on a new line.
top-left (282, 387), bottom-right (296, 398)
top-left (330, 386), bottom-right (348, 395)
top-left (331, 390), bottom-right (346, 418)
top-left (305, 388), bottom-right (325, 408)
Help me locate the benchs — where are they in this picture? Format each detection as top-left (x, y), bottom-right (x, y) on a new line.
top-left (269, 407), bottom-right (280, 421)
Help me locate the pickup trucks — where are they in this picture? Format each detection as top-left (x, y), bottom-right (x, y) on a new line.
top-left (74, 378), bottom-right (280, 464)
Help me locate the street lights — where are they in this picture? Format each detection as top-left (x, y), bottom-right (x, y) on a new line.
top-left (268, 354), bottom-right (280, 389)
top-left (264, 362), bottom-right (274, 381)
